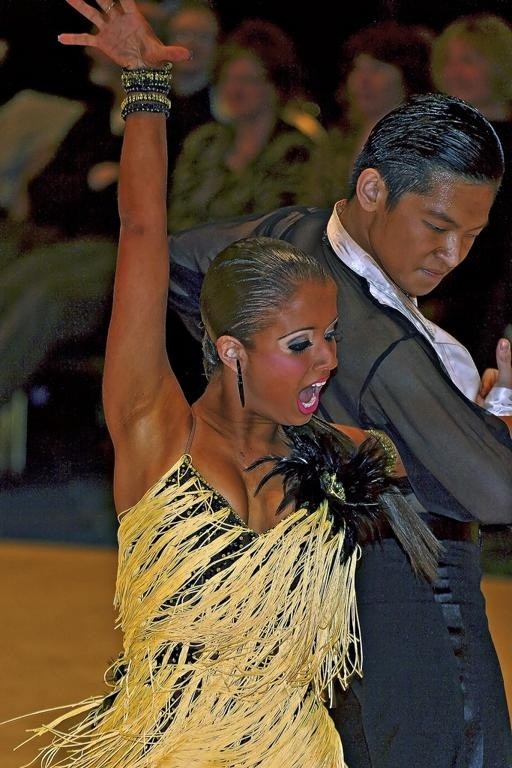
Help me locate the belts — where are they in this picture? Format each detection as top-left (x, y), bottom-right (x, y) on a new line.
top-left (360, 512), bottom-right (480, 543)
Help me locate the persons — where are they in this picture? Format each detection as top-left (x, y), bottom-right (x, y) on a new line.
top-left (170, 95), bottom-right (512, 768)
top-left (2, 0), bottom-right (512, 768)
top-left (0, 2), bottom-right (512, 482)
